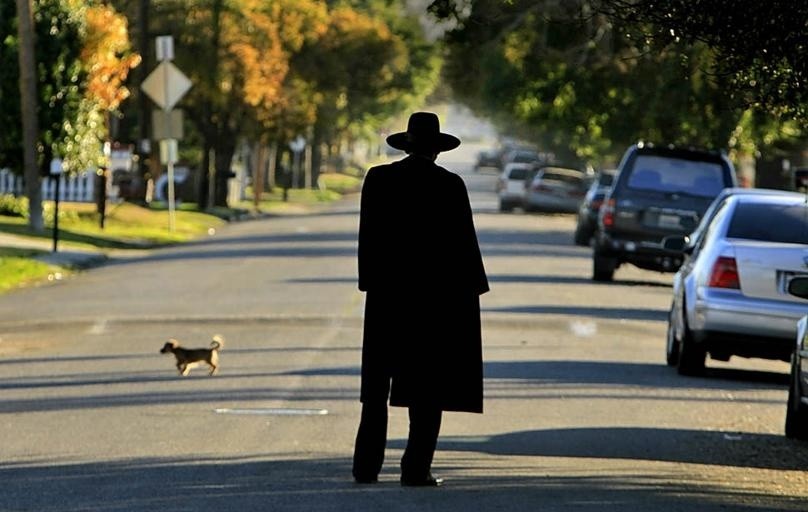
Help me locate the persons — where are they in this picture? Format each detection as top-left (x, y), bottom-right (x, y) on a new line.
top-left (353, 111), bottom-right (491, 487)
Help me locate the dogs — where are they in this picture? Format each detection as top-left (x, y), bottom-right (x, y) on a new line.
top-left (159, 334), bottom-right (223, 378)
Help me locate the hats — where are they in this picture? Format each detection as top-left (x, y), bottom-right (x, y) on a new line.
top-left (386, 112), bottom-right (460, 149)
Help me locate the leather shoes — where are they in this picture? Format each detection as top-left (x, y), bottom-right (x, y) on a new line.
top-left (430, 472), bottom-right (444, 487)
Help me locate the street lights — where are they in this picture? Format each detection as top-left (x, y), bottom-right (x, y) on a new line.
top-left (49, 157), bottom-right (63, 250)
top-left (287, 133), bottom-right (305, 189)
